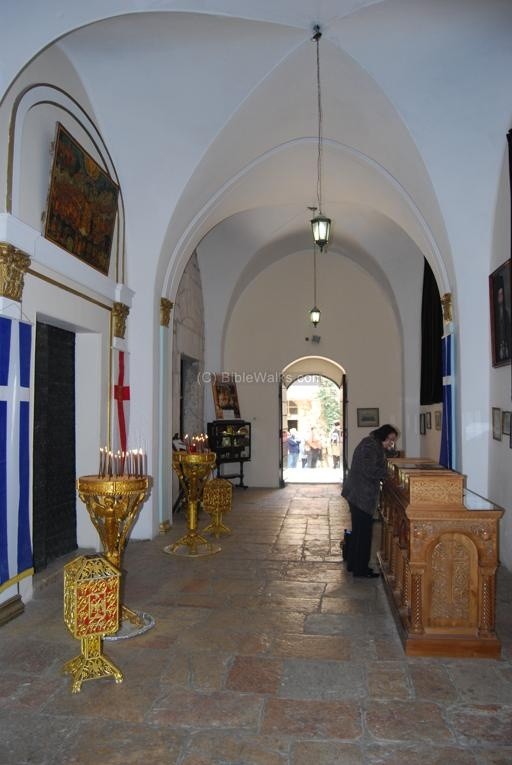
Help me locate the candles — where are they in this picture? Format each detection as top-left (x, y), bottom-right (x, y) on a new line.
top-left (97, 445), bottom-right (144, 479)
top-left (184, 432), bottom-right (209, 453)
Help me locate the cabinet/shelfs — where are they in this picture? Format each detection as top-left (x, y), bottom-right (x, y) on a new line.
top-left (206, 420), bottom-right (252, 489)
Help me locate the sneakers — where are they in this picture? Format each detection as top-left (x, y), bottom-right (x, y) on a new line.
top-left (346, 565), bottom-right (380, 579)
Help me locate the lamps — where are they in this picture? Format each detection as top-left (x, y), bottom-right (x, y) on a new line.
top-left (309, 23), bottom-right (332, 328)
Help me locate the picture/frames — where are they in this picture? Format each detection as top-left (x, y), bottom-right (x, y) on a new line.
top-left (492, 407), bottom-right (511, 441)
top-left (357, 407), bottom-right (379, 427)
top-left (420, 411), bottom-right (442, 435)
top-left (44, 120), bottom-right (121, 276)
top-left (487, 259), bottom-right (512, 369)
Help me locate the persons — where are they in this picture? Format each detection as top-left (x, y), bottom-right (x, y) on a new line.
top-left (288, 422), bottom-right (343, 468)
top-left (341, 424), bottom-right (398, 577)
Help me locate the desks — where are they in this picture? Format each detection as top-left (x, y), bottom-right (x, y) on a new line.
top-left (162, 451), bottom-right (223, 558)
top-left (76, 473), bottom-right (156, 642)
